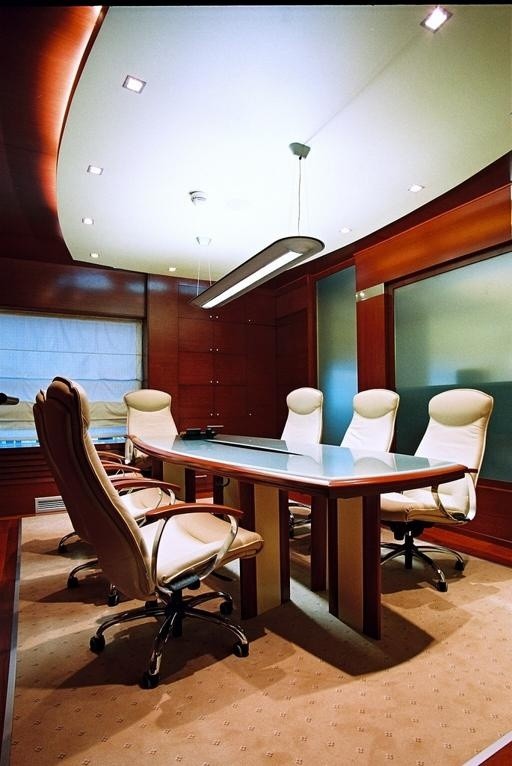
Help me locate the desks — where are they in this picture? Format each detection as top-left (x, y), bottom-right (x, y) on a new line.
top-left (123, 432), bottom-right (478, 640)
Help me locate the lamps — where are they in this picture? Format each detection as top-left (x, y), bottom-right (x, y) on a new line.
top-left (187, 144), bottom-right (325, 311)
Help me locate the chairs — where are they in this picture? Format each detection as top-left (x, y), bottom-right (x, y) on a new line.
top-left (280, 388), bottom-right (323, 539)
top-left (33, 377), bottom-right (188, 607)
top-left (339, 388), bottom-right (399, 453)
top-left (45, 379), bottom-right (263, 688)
top-left (380, 388), bottom-right (493, 592)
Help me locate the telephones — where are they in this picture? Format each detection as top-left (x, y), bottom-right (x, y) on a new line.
top-left (180, 428), bottom-right (215, 440)
